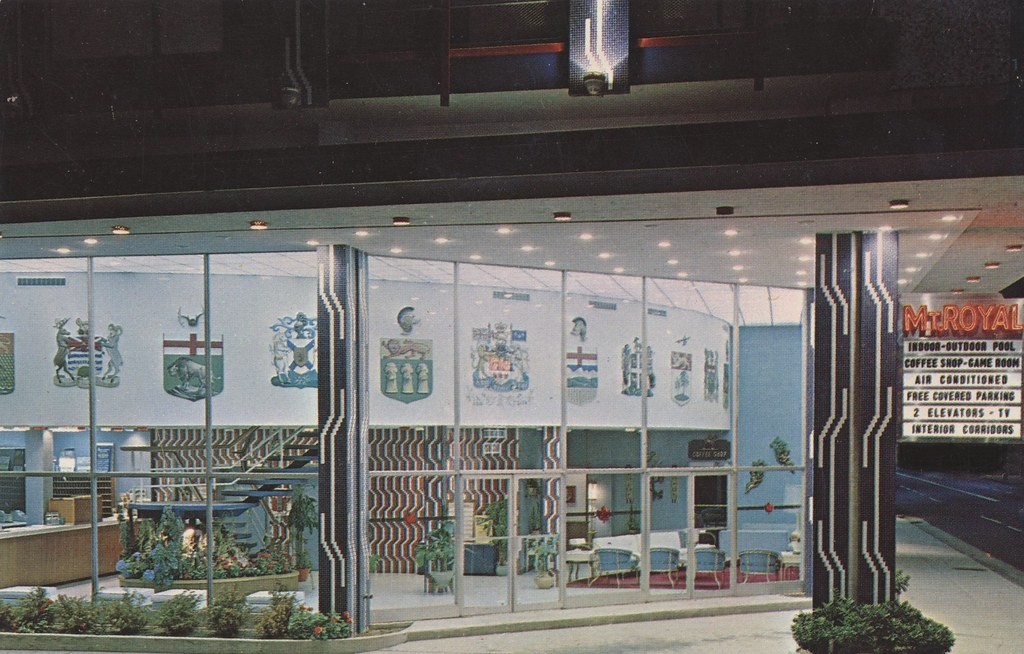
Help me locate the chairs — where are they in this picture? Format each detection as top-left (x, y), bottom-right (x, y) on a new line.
top-left (781, 549), bottom-right (803, 580)
top-left (649, 545), bottom-right (680, 590)
top-left (737, 548), bottom-right (778, 583)
top-left (588, 547), bottom-right (639, 587)
top-left (694, 547), bottom-right (727, 590)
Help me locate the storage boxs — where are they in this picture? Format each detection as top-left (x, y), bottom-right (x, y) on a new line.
top-left (48, 493), bottom-right (106, 526)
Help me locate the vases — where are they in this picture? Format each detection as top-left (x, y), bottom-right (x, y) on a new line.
top-left (115, 570), bottom-right (301, 594)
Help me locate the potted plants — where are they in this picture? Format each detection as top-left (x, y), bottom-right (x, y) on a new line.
top-left (289, 490), bottom-right (316, 582)
top-left (412, 527), bottom-right (454, 596)
top-left (476, 497), bottom-right (509, 578)
top-left (626, 516), bottom-right (639, 536)
top-left (528, 540), bottom-right (558, 588)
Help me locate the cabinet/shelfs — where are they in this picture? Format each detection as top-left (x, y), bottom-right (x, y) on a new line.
top-left (54, 476), bottom-right (116, 518)
